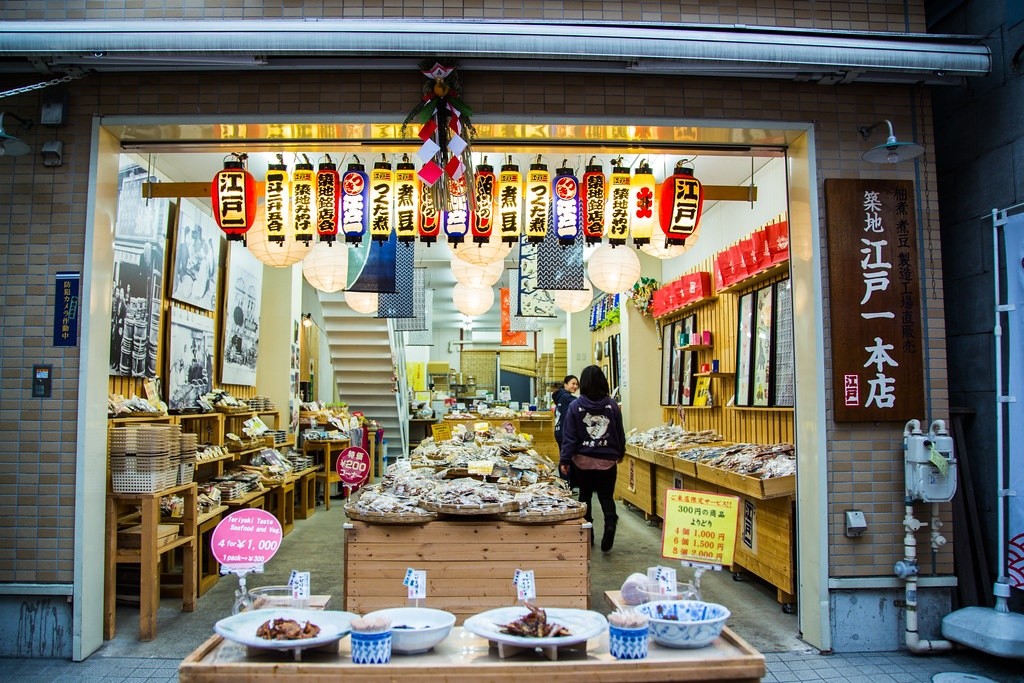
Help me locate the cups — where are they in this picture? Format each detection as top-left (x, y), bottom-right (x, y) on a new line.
top-left (608, 623), bottom-right (650, 660)
top-left (350, 630), bottom-right (392, 664)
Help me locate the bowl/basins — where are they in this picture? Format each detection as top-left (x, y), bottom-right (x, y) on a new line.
top-left (636, 581), bottom-right (697, 605)
top-left (362, 607), bottom-right (457, 655)
top-left (634, 600), bottom-right (731, 649)
top-left (248, 584), bottom-right (308, 611)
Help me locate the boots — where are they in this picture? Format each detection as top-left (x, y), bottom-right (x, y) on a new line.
top-left (601, 515), bottom-right (618, 551)
top-left (588, 519), bottom-right (594, 546)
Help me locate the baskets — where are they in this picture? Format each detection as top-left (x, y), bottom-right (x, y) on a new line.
top-left (237, 463), bottom-right (294, 478)
top-left (109, 424), bottom-right (199, 494)
top-left (213, 404), bottom-right (252, 413)
top-left (226, 438), bottom-right (266, 452)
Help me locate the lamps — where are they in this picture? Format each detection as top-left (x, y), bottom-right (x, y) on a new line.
top-left (859, 119), bottom-right (926, 165)
top-left (0, 109), bottom-right (32, 157)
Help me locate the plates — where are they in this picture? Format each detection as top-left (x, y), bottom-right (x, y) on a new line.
top-left (214, 608), bottom-right (361, 650)
top-left (464, 605), bottom-right (609, 647)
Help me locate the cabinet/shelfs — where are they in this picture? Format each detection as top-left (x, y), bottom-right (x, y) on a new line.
top-left (107, 403), bottom-right (382, 644)
top-left (343, 513), bottom-right (593, 614)
top-left (654, 258), bottom-right (796, 412)
top-left (624, 429), bottom-right (795, 500)
top-left (408, 417), bottom-right (438, 453)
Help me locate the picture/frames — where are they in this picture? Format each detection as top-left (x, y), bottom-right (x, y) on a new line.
top-left (659, 313), bottom-right (696, 405)
top-left (734, 277), bottom-right (794, 406)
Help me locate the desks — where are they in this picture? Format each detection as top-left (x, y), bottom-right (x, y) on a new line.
top-left (188, 591), bottom-right (766, 683)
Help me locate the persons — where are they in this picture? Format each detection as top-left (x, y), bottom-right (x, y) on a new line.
top-left (228, 334), bottom-right (256, 366)
top-left (111, 283), bottom-right (132, 370)
top-left (177, 343), bottom-right (213, 394)
top-left (561, 365), bottom-right (626, 551)
top-left (552, 375), bottom-right (580, 495)
top-left (176, 226), bottom-right (214, 283)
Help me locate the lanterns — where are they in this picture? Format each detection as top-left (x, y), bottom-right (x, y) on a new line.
top-left (659, 167), bottom-right (704, 245)
top-left (552, 168), bottom-right (580, 246)
top-left (631, 184), bottom-right (703, 259)
top-left (446, 204), bottom-right (515, 316)
top-left (582, 165), bottom-right (606, 243)
top-left (554, 279), bottom-right (593, 312)
top-left (211, 161), bottom-right (257, 241)
top-left (265, 164), bottom-right (289, 241)
top-left (630, 168), bottom-right (656, 244)
top-left (526, 164), bottom-right (549, 243)
top-left (248, 201), bottom-right (378, 314)
top-left (292, 163), bottom-right (522, 243)
top-left (589, 244), bottom-right (640, 296)
top-left (607, 167), bottom-right (632, 245)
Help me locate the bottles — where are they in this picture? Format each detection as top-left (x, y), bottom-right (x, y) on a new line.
top-left (449, 369), bottom-right (477, 398)
